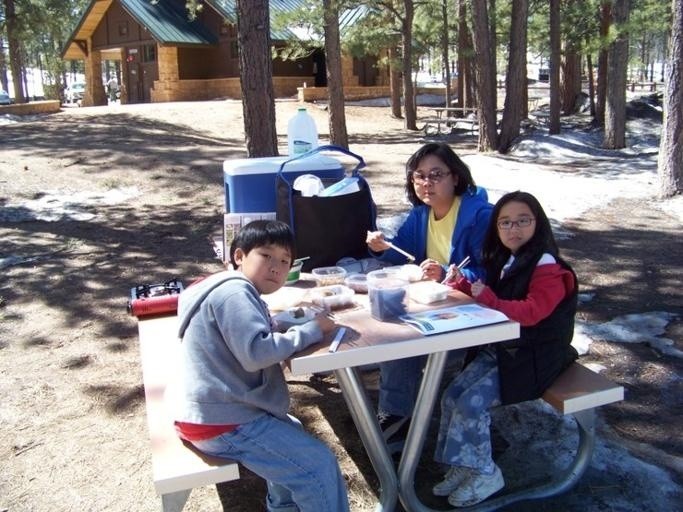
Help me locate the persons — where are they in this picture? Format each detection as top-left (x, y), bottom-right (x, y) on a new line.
top-left (430, 189), bottom-right (579, 507)
top-left (170, 216), bottom-right (348, 511)
top-left (105, 75), bottom-right (117, 102)
top-left (364, 141), bottom-right (498, 455)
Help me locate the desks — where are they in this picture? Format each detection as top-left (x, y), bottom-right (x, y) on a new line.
top-left (249, 267), bottom-right (522, 512)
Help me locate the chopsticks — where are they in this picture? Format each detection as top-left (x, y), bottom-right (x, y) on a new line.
top-left (440, 255), bottom-right (471, 285)
top-left (367, 230), bottom-right (416, 262)
top-left (308, 303), bottom-right (336, 319)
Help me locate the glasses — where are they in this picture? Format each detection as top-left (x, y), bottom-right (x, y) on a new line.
top-left (409, 168), bottom-right (452, 185)
top-left (497, 215), bottom-right (533, 230)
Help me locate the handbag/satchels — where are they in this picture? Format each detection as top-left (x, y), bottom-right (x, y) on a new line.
top-left (274, 144), bottom-right (378, 273)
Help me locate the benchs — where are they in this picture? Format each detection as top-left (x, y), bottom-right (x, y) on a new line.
top-left (139, 295), bottom-right (244, 512)
top-left (504, 351), bottom-right (625, 500)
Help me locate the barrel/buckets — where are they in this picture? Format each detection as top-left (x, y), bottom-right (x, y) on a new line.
top-left (286, 108), bottom-right (320, 160)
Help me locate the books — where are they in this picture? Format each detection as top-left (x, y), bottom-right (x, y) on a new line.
top-left (395, 303), bottom-right (510, 337)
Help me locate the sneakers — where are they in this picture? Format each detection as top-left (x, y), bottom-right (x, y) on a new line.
top-left (447, 462), bottom-right (505, 507)
top-left (433, 464), bottom-right (469, 496)
top-left (373, 413), bottom-right (411, 456)
